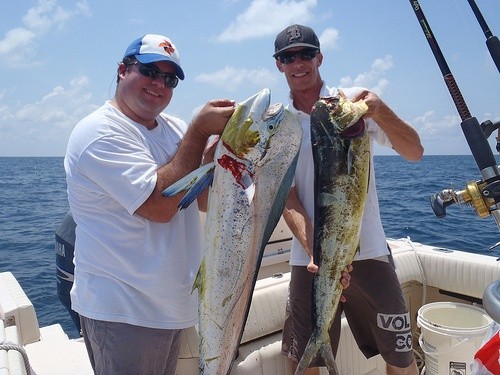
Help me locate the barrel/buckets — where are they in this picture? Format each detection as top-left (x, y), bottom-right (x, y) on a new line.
top-left (417, 302), bottom-right (494, 375)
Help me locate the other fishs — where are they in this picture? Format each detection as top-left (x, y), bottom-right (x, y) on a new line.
top-left (296, 98), bottom-right (372, 375)
top-left (166, 87), bottom-right (304, 375)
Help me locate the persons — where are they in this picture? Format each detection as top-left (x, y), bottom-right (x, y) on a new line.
top-left (272, 25), bottom-right (425, 375)
top-left (63, 34), bottom-right (237, 375)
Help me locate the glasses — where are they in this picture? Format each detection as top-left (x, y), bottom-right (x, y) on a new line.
top-left (275, 48), bottom-right (318, 66)
top-left (123, 60), bottom-right (178, 90)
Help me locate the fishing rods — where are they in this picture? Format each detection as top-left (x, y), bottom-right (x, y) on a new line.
top-left (409, 0), bottom-right (500, 255)
top-left (468, 0), bottom-right (500, 73)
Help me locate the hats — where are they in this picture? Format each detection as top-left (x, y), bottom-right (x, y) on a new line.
top-left (124, 34), bottom-right (193, 79)
top-left (271, 24), bottom-right (323, 56)
